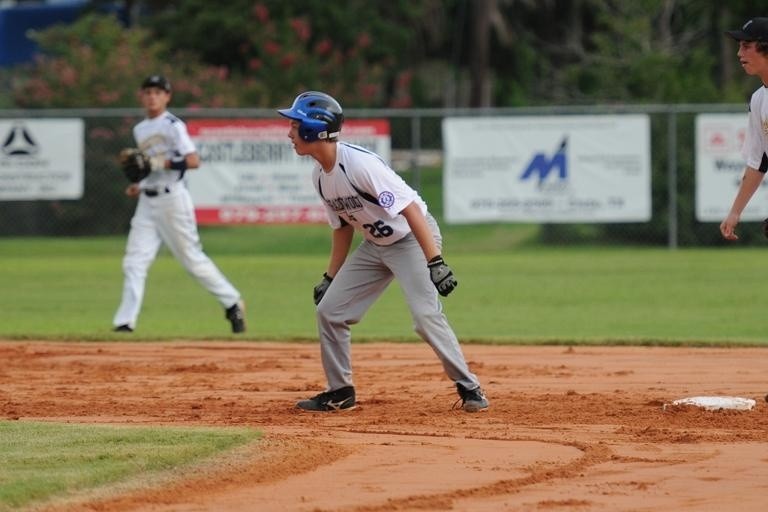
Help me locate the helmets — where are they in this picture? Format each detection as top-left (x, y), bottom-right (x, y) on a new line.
top-left (277, 90), bottom-right (345, 143)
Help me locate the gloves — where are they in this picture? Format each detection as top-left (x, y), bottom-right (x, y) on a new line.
top-left (428, 254), bottom-right (457, 297)
top-left (314, 273), bottom-right (332, 305)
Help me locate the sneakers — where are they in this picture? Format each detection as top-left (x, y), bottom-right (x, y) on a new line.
top-left (456, 382), bottom-right (488, 413)
top-left (296, 386), bottom-right (355, 411)
top-left (226, 299), bottom-right (246, 332)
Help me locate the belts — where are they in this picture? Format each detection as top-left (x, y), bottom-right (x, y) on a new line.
top-left (144, 187), bottom-right (169, 197)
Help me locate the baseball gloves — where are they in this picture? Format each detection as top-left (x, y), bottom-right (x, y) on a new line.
top-left (121, 147), bottom-right (150, 184)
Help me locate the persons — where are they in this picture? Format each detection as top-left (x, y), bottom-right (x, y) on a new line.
top-left (111, 73), bottom-right (249, 332)
top-left (275, 89), bottom-right (492, 413)
top-left (717, 14), bottom-right (768, 241)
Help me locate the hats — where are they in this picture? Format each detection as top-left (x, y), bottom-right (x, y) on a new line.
top-left (723, 16), bottom-right (768, 42)
top-left (141, 75), bottom-right (171, 93)
